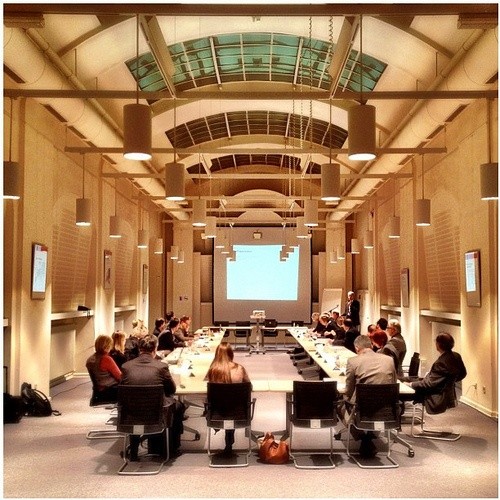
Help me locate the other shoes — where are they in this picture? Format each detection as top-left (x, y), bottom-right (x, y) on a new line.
top-left (358, 438), bottom-right (378, 458)
top-left (222, 447), bottom-right (233, 457)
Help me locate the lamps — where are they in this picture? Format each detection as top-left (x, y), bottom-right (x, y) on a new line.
top-left (3, 3), bottom-right (499, 264)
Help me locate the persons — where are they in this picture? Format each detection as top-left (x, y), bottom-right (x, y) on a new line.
top-left (203, 341), bottom-right (253, 453)
top-left (85, 311), bottom-right (200, 460)
top-left (306, 291), bottom-right (467, 457)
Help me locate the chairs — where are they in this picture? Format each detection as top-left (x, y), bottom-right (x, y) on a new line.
top-left (84, 319), bottom-right (462, 475)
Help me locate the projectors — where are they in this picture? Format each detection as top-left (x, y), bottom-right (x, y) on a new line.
top-left (250, 314), bottom-right (266, 323)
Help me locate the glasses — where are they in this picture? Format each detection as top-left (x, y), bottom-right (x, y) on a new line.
top-left (386, 327), bottom-right (391, 329)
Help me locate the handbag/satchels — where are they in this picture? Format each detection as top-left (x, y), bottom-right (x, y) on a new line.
top-left (258, 432), bottom-right (289, 465)
top-left (78, 305), bottom-right (91, 320)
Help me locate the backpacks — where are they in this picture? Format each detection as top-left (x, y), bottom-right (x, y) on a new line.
top-left (3, 392), bottom-right (24, 424)
top-left (21, 382), bottom-right (61, 417)
